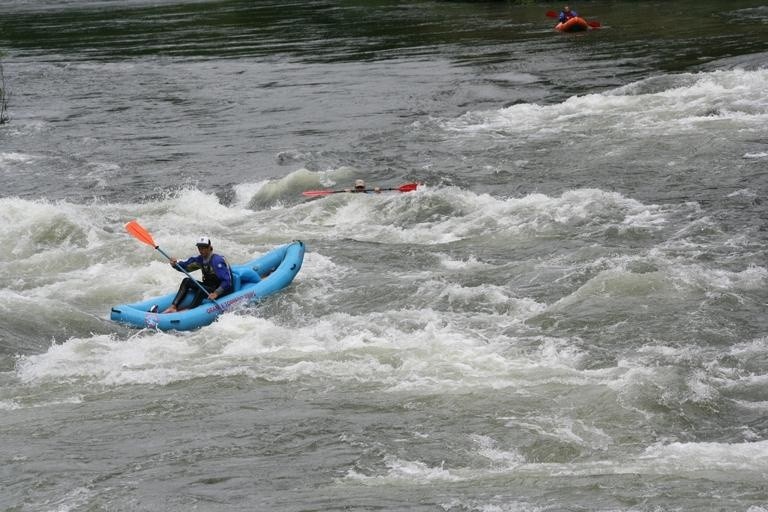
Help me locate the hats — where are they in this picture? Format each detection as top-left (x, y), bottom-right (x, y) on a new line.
top-left (355, 180), bottom-right (364, 187)
top-left (195, 238), bottom-right (210, 247)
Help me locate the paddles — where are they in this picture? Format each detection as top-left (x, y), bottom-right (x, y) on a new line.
top-left (545, 10), bottom-right (600, 27)
top-left (125, 219), bottom-right (226, 313)
top-left (302, 183), bottom-right (418, 195)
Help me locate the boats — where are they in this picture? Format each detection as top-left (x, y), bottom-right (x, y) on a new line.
top-left (106, 238), bottom-right (307, 333)
top-left (555, 17), bottom-right (591, 35)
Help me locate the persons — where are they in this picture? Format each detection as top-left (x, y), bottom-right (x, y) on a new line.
top-left (162, 236), bottom-right (234, 314)
top-left (556, 6), bottom-right (577, 28)
top-left (344, 179), bottom-right (380, 195)
